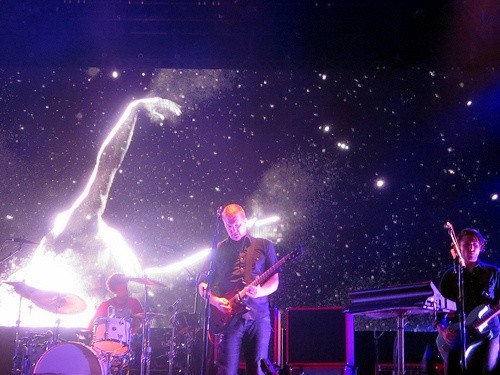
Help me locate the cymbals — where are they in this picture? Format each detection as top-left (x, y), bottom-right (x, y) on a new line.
top-left (5, 281), bottom-right (87, 315)
top-left (132, 311), bottom-right (164, 319)
top-left (125, 278), bottom-right (167, 287)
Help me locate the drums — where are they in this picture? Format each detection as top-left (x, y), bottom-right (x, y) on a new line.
top-left (22, 344), bottom-right (52, 375)
top-left (33, 341), bottom-right (111, 375)
top-left (92, 316), bottom-right (131, 356)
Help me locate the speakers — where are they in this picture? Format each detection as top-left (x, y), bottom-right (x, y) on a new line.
top-left (286, 306), bottom-right (354, 367)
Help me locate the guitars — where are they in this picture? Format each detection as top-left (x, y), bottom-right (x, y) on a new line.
top-left (205, 245), bottom-right (304, 332)
top-left (436, 301), bottom-right (500, 363)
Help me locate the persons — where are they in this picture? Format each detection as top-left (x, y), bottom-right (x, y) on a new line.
top-left (7, 96), bottom-right (287, 313)
top-left (422, 228), bottom-right (500, 375)
top-left (86, 273), bottom-right (145, 337)
top-left (197, 203), bottom-right (279, 375)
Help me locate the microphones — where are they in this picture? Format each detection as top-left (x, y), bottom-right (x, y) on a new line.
top-left (11, 238), bottom-right (41, 245)
